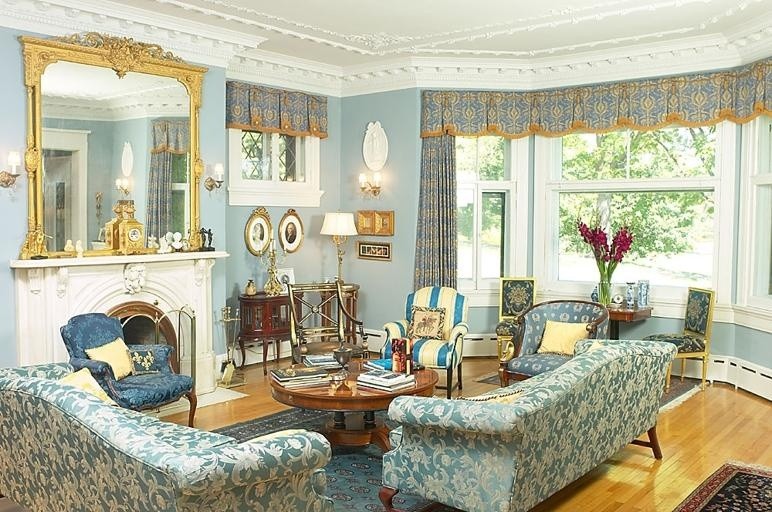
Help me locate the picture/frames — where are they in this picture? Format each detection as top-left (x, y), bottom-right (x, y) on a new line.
top-left (278, 208), bottom-right (304, 254)
top-left (244, 206), bottom-right (274, 258)
top-left (355, 208), bottom-right (376, 235)
top-left (276, 267), bottom-right (295, 294)
top-left (374, 210), bottom-right (395, 236)
top-left (354, 240), bottom-right (392, 263)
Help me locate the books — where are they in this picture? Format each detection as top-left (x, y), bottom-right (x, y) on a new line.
top-left (356, 338), bottom-right (425, 391)
top-left (271, 355), bottom-right (349, 390)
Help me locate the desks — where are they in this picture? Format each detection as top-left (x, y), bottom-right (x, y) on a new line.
top-left (235, 290), bottom-right (304, 375)
top-left (597, 305), bottom-right (654, 339)
top-left (267, 349), bottom-right (439, 459)
top-left (321, 282), bottom-right (358, 343)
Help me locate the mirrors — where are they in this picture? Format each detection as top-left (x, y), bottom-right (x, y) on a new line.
top-left (17, 30), bottom-right (210, 257)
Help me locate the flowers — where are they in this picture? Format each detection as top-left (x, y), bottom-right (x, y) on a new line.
top-left (576, 219), bottom-right (632, 302)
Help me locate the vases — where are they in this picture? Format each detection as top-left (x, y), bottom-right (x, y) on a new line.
top-left (596, 281), bottom-right (612, 310)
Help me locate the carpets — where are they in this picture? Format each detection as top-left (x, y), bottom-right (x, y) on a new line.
top-left (670, 454), bottom-right (772, 511)
top-left (474, 367), bottom-right (706, 415)
top-left (211, 401), bottom-right (459, 511)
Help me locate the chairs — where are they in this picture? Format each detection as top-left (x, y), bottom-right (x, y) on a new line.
top-left (644, 287), bottom-right (716, 394)
top-left (58, 313), bottom-right (198, 430)
top-left (283, 285), bottom-right (364, 413)
top-left (0, 359), bottom-right (335, 511)
top-left (496, 298), bottom-right (610, 393)
top-left (380, 339), bottom-right (677, 512)
top-left (390, 287), bottom-right (469, 401)
top-left (497, 279), bottom-right (536, 360)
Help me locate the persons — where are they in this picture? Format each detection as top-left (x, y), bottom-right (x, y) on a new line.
top-left (252, 224), bottom-right (265, 245)
top-left (285, 222), bottom-right (297, 243)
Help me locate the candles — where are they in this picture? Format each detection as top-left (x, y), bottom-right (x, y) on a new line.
top-left (257, 238), bottom-right (287, 253)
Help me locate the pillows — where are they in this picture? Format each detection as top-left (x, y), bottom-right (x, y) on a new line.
top-left (60, 366), bottom-right (117, 406)
top-left (82, 336), bottom-right (132, 381)
top-left (537, 317), bottom-right (590, 354)
top-left (407, 303), bottom-right (447, 340)
top-left (126, 347), bottom-right (160, 375)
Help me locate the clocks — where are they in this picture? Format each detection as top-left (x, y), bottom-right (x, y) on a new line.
top-left (103, 203), bottom-right (120, 250)
top-left (118, 199), bottom-right (145, 255)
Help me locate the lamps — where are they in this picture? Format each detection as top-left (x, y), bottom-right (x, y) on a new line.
top-left (204, 162), bottom-right (225, 192)
top-left (358, 171), bottom-right (382, 196)
top-left (321, 212), bottom-right (358, 285)
top-left (0, 149), bottom-right (20, 189)
top-left (114, 177), bottom-right (130, 197)
